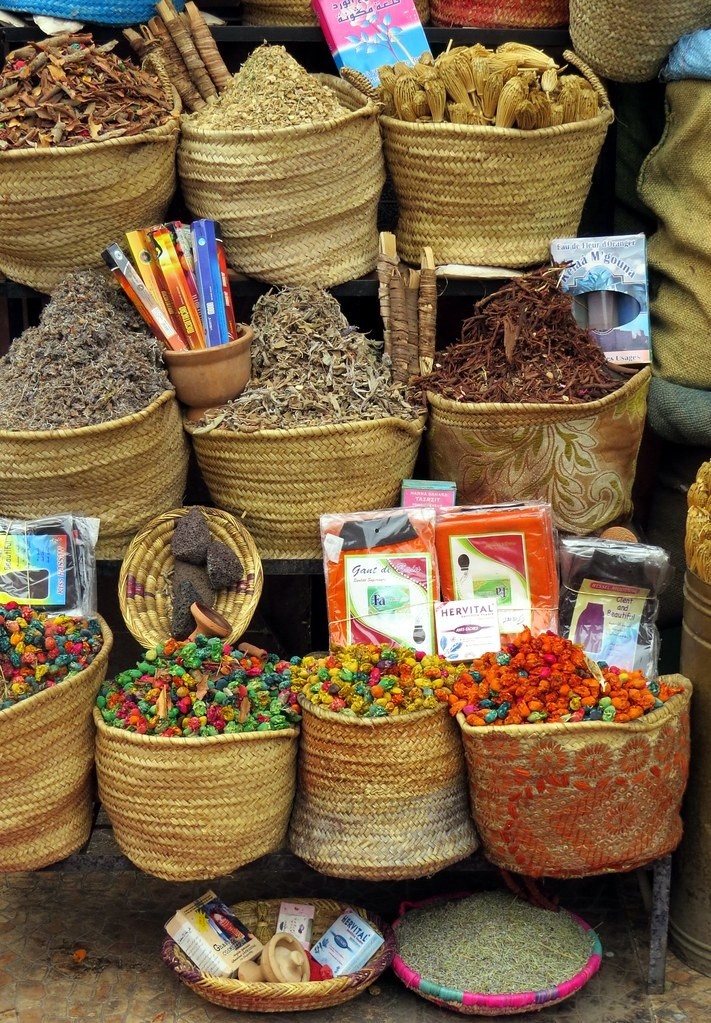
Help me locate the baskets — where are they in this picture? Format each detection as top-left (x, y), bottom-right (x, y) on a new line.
top-left (0, 0), bottom-right (709, 879)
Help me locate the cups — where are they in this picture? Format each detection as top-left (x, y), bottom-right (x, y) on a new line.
top-left (238, 932), bottom-right (310, 983)
